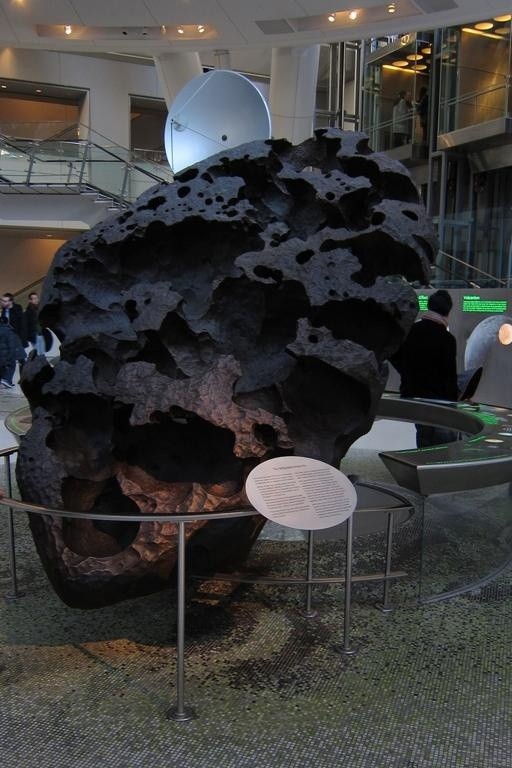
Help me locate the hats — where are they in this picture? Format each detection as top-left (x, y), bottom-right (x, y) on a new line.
top-left (396, 89), bottom-right (407, 96)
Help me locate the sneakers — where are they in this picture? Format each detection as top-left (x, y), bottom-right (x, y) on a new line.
top-left (1, 380), bottom-right (15, 388)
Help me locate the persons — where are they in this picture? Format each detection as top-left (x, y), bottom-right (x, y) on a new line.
top-left (0, 293), bottom-right (46, 388)
top-left (393, 87), bottom-right (428, 147)
top-left (388, 290), bottom-right (462, 448)
